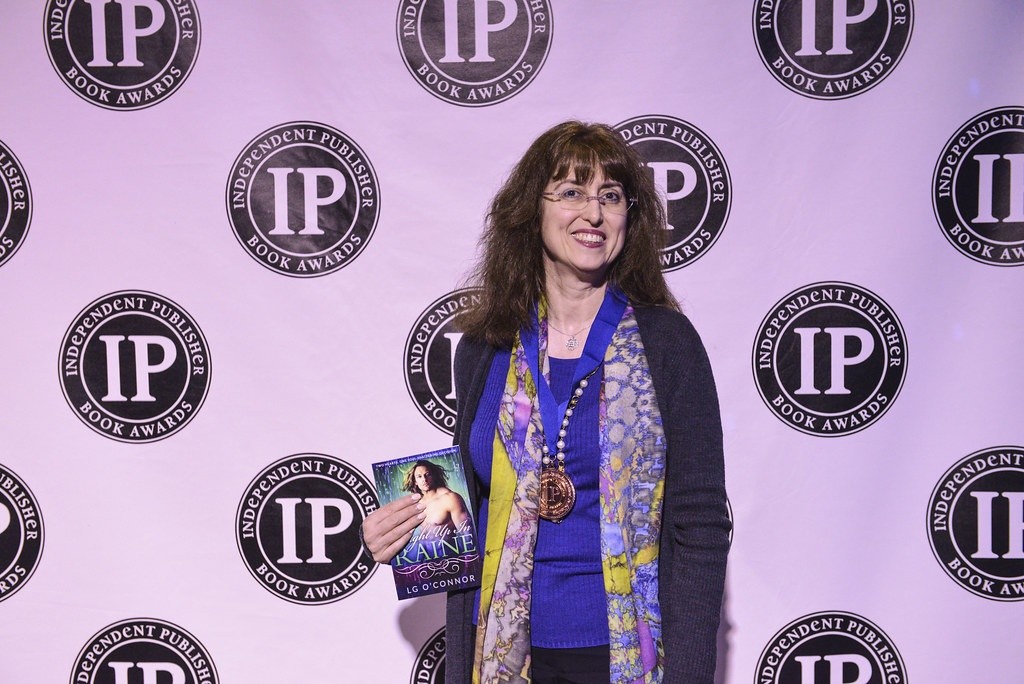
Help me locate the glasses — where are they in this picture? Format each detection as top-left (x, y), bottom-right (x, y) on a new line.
top-left (541, 188), bottom-right (638, 215)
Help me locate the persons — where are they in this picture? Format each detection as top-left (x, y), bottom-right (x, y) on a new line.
top-left (395, 457), bottom-right (473, 561)
top-left (358, 118), bottom-right (731, 684)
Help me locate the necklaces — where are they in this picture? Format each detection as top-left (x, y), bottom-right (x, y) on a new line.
top-left (536, 366), bottom-right (602, 527)
top-left (545, 316), bottom-right (592, 351)
